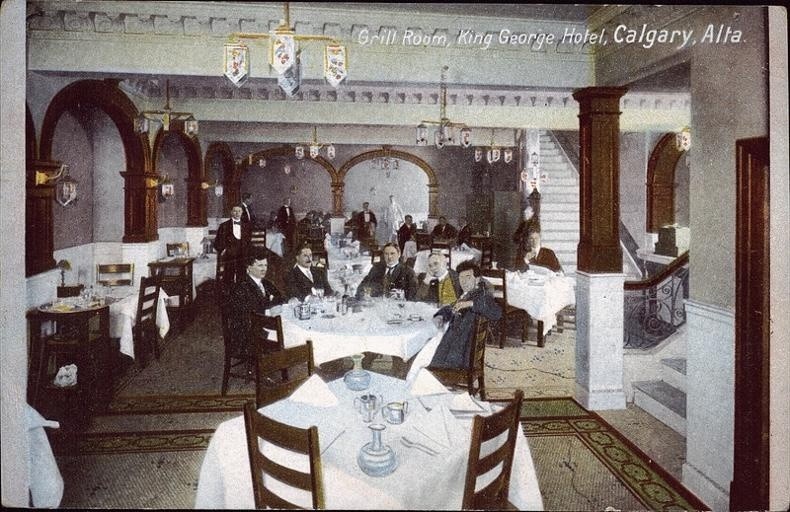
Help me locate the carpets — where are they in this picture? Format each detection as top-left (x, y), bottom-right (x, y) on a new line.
top-left (43, 393), bottom-right (710, 512)
top-left (87, 297), bottom-right (258, 417)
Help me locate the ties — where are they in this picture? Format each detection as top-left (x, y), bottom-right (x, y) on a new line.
top-left (386, 267), bottom-right (393, 279)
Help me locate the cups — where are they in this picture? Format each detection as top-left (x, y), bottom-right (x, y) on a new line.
top-left (354, 393), bottom-right (381, 420)
top-left (294, 284), bottom-right (437, 323)
top-left (506, 270), bottom-right (564, 288)
top-left (382, 401), bottom-right (409, 424)
top-left (63, 281), bottom-right (107, 309)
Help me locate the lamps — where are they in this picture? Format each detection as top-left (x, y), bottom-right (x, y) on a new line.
top-left (132, 80), bottom-right (199, 142)
top-left (295, 127), bottom-right (337, 162)
top-left (221, 2), bottom-right (349, 97)
top-left (415, 88), bottom-right (472, 151)
top-left (148, 170), bottom-right (175, 199)
top-left (474, 130), bottom-right (513, 165)
top-left (199, 177), bottom-right (223, 197)
top-left (33, 163), bottom-right (80, 208)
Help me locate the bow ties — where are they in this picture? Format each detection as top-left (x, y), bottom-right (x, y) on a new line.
top-left (234, 221), bottom-right (240, 224)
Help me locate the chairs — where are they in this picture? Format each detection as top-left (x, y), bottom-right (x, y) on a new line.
top-left (24, 209), bottom-right (579, 512)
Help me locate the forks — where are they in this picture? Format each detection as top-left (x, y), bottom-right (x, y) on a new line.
top-left (400, 437), bottom-right (440, 459)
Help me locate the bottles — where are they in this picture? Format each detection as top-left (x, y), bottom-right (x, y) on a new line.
top-left (358, 423), bottom-right (398, 479)
top-left (344, 354), bottom-right (372, 391)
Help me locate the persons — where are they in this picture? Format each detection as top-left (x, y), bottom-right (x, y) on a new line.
top-left (213, 193), bottom-right (566, 275)
top-left (413, 251), bottom-right (465, 304)
top-left (227, 249), bottom-right (288, 352)
top-left (354, 242), bottom-right (419, 374)
top-left (281, 244), bottom-right (334, 302)
top-left (430, 260), bottom-right (503, 370)
